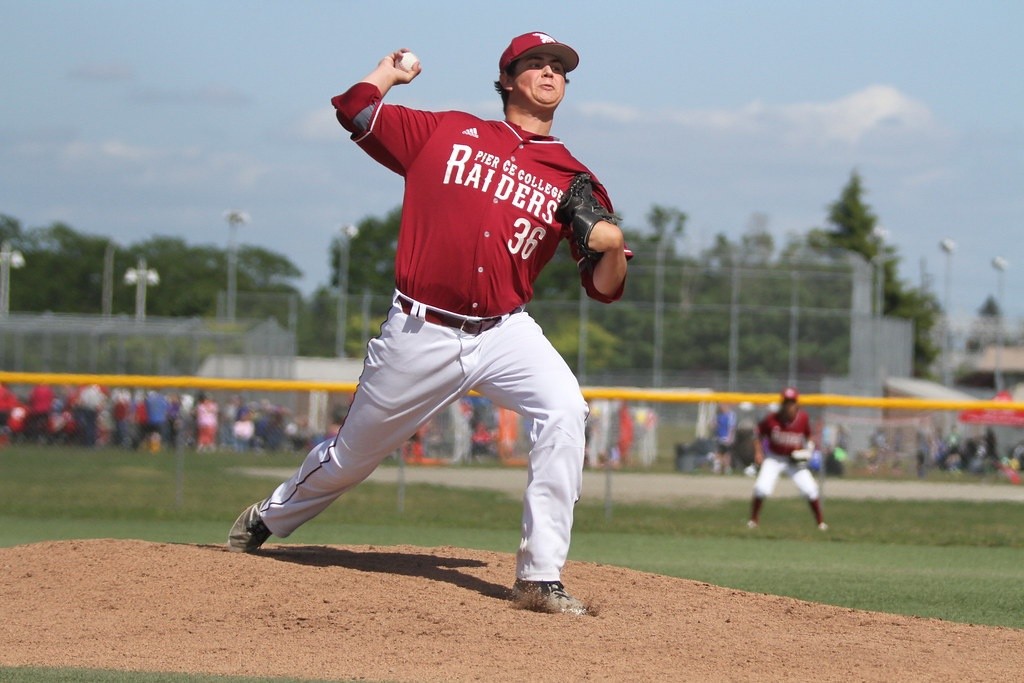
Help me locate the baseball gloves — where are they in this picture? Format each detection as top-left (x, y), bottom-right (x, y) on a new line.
top-left (554, 172), bottom-right (622, 262)
top-left (790, 448), bottom-right (811, 467)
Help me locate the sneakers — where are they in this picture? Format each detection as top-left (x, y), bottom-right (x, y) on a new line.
top-left (227, 499), bottom-right (268, 551)
top-left (514, 578), bottom-right (586, 614)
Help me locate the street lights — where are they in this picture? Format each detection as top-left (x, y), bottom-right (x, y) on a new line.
top-left (222, 206), bottom-right (249, 319)
top-left (987, 256), bottom-right (1008, 391)
top-left (0, 242), bottom-right (25, 317)
top-left (939, 239), bottom-right (961, 387)
top-left (124, 258), bottom-right (161, 325)
top-left (331, 219), bottom-right (359, 359)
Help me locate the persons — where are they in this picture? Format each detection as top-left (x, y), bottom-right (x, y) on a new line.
top-left (227, 33), bottom-right (634, 614)
top-left (744, 426), bottom-right (770, 476)
top-left (712, 402), bottom-right (737, 474)
top-left (945, 425), bottom-right (1024, 477)
top-left (809, 445), bottom-right (848, 475)
top-left (745, 387), bottom-right (828, 530)
top-left (0, 381), bottom-right (500, 462)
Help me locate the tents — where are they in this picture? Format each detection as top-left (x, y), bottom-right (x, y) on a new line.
top-left (959, 391), bottom-right (1024, 428)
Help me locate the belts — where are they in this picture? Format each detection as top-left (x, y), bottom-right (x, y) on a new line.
top-left (398, 296), bottom-right (519, 334)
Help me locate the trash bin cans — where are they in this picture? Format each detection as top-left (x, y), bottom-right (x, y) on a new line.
top-left (675, 443), bottom-right (696, 472)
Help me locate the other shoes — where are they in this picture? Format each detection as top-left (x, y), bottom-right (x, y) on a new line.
top-left (747, 521), bottom-right (755, 528)
top-left (819, 523), bottom-right (826, 529)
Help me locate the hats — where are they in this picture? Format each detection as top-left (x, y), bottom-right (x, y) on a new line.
top-left (499, 32), bottom-right (579, 75)
top-left (782, 387), bottom-right (798, 401)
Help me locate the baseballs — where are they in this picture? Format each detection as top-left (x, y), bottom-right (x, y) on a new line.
top-left (395, 51), bottom-right (417, 71)
